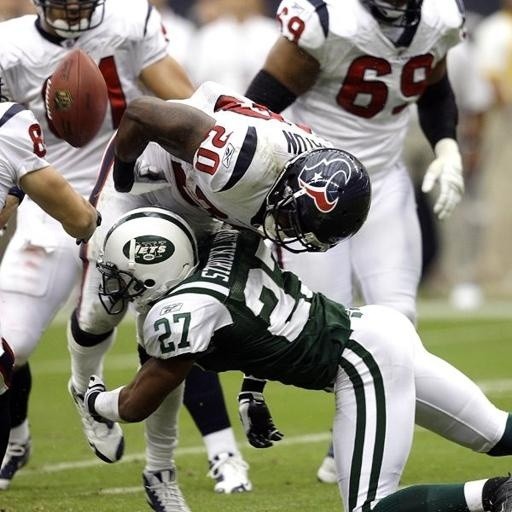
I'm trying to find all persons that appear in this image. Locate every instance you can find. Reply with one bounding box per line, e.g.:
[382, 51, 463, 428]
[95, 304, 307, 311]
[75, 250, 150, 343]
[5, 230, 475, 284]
[0, 99, 103, 472]
[69, 79, 373, 465]
[0, 0, 512, 316]
[240, 0, 467, 487]
[0, 0, 199, 491]
[87, 205, 512, 512]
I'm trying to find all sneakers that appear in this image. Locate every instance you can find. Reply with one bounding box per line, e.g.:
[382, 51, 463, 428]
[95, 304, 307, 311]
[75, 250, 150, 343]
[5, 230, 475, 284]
[315, 457, 341, 487]
[205, 448, 254, 496]
[488, 473, 511, 511]
[140, 462, 192, 512]
[65, 371, 127, 465]
[0, 430, 34, 492]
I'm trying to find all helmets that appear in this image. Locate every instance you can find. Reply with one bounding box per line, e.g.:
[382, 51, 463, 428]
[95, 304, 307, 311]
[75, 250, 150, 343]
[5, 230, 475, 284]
[95, 205, 202, 313]
[262, 145, 375, 257]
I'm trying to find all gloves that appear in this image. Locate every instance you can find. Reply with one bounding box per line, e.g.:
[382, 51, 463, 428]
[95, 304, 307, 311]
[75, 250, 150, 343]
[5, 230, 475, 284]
[421, 137, 468, 223]
[236, 378, 285, 449]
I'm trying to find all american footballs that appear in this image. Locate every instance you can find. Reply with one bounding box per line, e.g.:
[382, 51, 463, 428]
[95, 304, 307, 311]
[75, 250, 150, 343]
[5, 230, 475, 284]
[46, 49, 105, 147]
